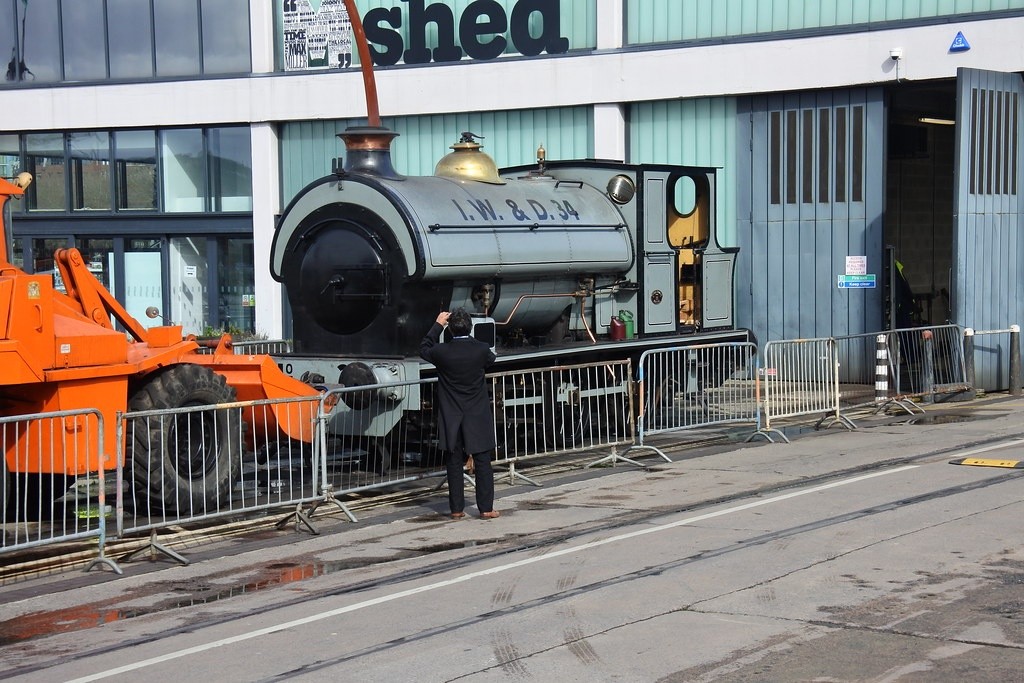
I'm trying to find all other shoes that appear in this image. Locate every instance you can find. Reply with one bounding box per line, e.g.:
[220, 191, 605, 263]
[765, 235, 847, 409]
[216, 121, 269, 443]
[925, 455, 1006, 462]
[480, 509, 499, 520]
[450, 512, 465, 519]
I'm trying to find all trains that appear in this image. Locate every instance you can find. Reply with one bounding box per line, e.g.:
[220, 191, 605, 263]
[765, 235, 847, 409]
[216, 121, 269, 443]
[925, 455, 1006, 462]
[260, 125, 757, 477]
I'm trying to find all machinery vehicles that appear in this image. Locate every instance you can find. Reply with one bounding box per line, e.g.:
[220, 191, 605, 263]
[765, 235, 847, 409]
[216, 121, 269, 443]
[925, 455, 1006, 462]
[0, 172, 346, 514]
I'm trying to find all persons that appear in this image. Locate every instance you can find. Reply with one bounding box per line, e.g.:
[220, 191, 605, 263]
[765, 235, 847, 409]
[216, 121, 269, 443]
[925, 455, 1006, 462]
[416, 305, 502, 523]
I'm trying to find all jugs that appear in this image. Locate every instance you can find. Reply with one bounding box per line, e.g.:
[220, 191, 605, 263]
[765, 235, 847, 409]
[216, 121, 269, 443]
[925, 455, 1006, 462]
[619, 310, 634, 339]
[611, 316, 626, 340]
